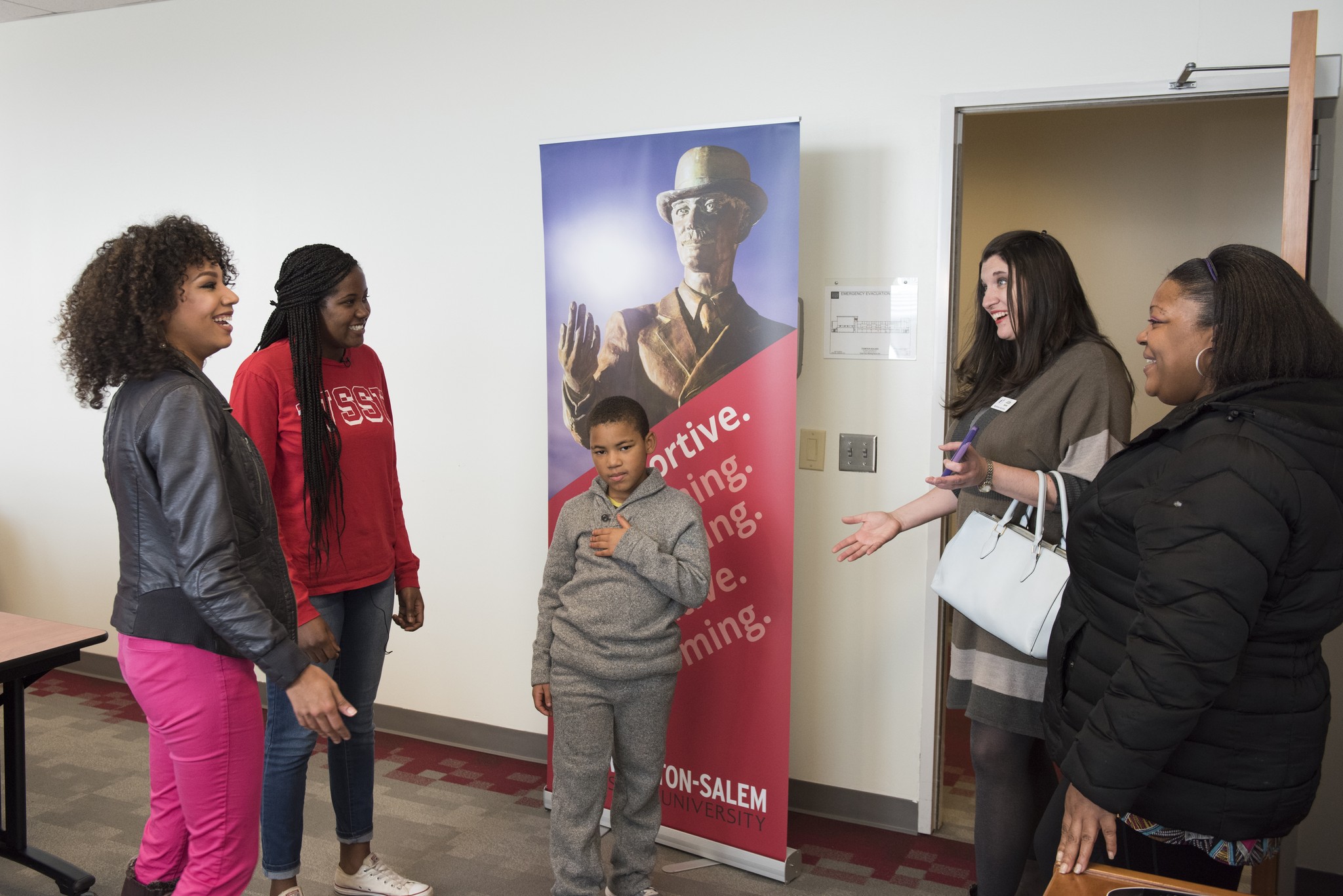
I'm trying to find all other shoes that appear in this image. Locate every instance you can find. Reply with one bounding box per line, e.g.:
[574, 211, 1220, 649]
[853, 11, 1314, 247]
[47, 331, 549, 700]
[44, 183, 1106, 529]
[605, 885, 660, 896]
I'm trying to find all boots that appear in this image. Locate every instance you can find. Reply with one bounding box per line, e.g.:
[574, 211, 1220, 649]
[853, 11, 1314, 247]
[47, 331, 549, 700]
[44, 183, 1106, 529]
[121, 856, 180, 896]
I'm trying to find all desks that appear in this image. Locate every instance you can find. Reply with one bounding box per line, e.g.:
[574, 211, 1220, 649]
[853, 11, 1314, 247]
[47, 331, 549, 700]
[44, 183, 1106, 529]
[0, 611, 109, 896]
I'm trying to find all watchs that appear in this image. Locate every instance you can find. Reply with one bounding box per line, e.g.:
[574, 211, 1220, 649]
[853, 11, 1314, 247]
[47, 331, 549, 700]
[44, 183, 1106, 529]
[976, 457, 994, 493]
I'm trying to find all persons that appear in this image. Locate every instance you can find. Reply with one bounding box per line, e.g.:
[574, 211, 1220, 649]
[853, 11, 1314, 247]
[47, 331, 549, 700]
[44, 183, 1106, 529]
[230, 245, 438, 896]
[531, 394, 710, 896]
[556, 147, 797, 451]
[832, 231, 1139, 896]
[1012, 243, 1343, 896]
[58, 215, 358, 896]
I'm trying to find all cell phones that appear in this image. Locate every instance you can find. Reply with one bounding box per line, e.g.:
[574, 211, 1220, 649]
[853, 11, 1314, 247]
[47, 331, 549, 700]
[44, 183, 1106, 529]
[941, 426, 979, 477]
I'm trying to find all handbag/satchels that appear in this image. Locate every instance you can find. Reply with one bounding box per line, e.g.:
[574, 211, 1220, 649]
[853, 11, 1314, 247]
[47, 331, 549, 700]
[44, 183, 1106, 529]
[931, 469, 1070, 660]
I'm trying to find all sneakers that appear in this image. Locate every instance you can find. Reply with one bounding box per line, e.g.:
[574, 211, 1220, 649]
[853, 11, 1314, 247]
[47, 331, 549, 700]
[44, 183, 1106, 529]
[334, 852, 434, 895]
[277, 886, 304, 896]
